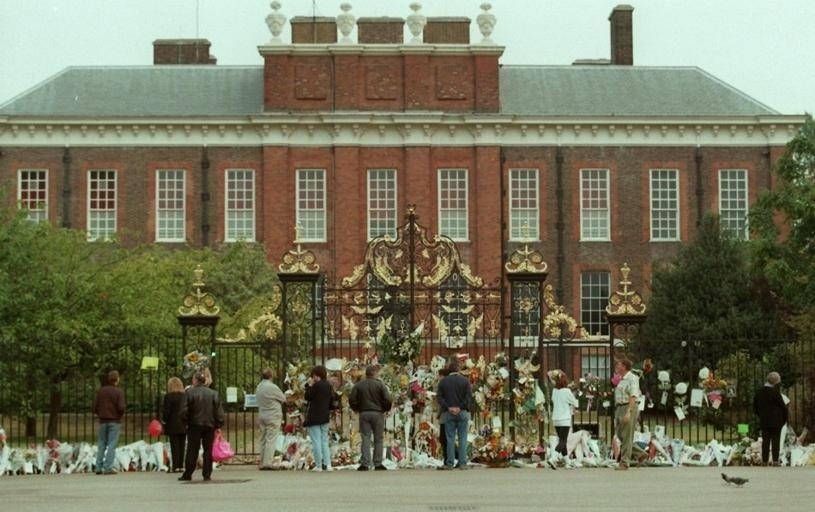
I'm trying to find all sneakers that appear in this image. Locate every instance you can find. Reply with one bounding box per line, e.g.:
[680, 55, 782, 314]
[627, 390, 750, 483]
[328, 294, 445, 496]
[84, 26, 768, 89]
[763, 462, 781, 466]
[311, 465, 331, 471]
[358, 465, 387, 470]
[259, 467, 275, 470]
[615, 453, 648, 470]
[96, 468, 118, 474]
[437, 464, 472, 469]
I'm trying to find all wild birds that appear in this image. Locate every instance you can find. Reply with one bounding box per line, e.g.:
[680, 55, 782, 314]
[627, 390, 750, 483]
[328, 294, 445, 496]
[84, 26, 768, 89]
[721, 473, 732, 485]
[729, 477, 749, 488]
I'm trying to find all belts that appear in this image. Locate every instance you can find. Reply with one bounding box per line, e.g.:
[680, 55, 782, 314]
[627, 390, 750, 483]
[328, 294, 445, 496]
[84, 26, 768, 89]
[617, 402, 630, 405]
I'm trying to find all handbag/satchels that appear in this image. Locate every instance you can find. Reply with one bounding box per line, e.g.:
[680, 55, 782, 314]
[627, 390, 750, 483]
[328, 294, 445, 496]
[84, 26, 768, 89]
[329, 391, 339, 409]
[212, 430, 234, 461]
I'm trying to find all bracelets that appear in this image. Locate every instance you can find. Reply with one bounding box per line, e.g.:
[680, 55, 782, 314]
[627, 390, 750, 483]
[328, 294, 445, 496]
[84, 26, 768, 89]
[627, 407, 632, 412]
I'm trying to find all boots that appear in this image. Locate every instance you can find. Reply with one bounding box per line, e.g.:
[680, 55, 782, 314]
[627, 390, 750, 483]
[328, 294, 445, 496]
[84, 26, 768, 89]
[548, 451, 574, 469]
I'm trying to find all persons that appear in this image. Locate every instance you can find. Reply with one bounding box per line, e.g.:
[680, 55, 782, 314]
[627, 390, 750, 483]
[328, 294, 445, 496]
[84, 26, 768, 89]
[174, 372, 224, 480]
[302, 366, 333, 470]
[159, 375, 186, 472]
[349, 365, 393, 471]
[433, 368, 462, 469]
[752, 371, 788, 466]
[609, 359, 643, 472]
[92, 369, 125, 475]
[254, 368, 286, 471]
[437, 362, 474, 470]
[547, 376, 580, 471]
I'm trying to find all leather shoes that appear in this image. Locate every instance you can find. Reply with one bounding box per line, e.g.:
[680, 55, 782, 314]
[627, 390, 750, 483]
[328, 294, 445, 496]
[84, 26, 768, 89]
[166, 467, 211, 481]
